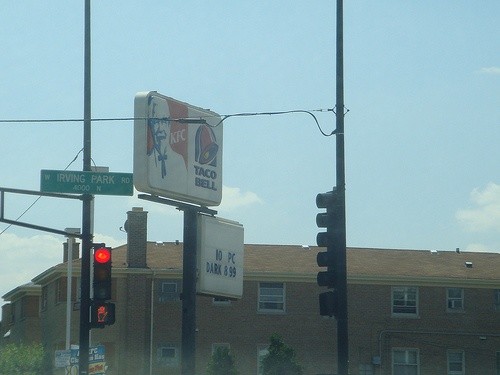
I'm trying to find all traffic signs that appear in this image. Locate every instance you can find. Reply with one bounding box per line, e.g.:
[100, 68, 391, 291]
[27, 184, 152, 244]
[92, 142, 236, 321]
[40, 169, 134, 196]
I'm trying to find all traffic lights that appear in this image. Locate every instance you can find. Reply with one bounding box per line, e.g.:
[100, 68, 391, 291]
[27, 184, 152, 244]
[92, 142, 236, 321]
[92, 245, 111, 300]
[316, 186, 349, 318]
[89, 303, 116, 326]
